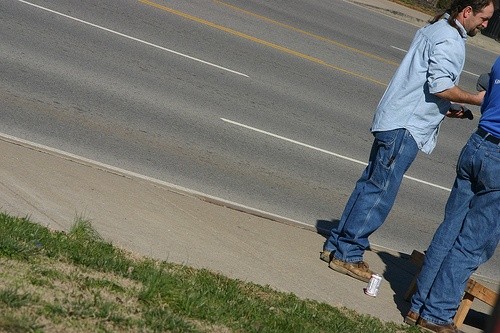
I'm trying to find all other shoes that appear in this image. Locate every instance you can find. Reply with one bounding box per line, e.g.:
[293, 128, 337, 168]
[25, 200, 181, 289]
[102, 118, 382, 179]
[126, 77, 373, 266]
[320, 248, 332, 263]
[329, 255, 374, 283]
[403, 309, 421, 327]
[416, 316, 464, 333]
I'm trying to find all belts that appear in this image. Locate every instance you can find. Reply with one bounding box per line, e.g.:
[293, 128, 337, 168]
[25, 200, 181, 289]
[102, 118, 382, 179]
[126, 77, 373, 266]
[476, 126, 500, 144]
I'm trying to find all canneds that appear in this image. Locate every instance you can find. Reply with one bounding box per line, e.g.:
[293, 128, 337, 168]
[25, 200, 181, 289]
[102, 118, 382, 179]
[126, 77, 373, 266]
[365, 273, 383, 297]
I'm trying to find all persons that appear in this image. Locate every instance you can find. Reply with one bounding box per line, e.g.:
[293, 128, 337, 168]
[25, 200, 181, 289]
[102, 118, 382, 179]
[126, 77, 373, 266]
[319, 0, 495, 284]
[404, 53, 500, 333]
